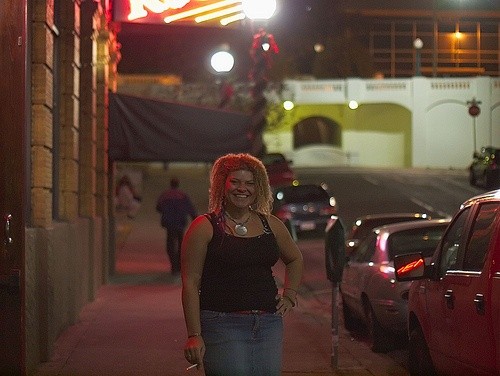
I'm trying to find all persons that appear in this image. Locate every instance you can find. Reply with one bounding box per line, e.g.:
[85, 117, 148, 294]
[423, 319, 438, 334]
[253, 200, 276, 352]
[181, 153, 304, 376]
[156, 176, 197, 274]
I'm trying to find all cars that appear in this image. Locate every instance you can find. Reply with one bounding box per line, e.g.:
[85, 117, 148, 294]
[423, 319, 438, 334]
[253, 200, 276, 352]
[394, 189, 500, 375]
[468, 146, 500, 188]
[260, 154, 339, 241]
[340, 214, 454, 353]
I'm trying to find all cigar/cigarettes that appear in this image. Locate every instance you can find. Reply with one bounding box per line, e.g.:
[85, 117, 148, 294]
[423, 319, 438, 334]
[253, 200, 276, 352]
[186, 364, 197, 371]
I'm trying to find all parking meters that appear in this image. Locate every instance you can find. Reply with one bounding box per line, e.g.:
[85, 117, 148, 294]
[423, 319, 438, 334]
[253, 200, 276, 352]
[324, 215, 346, 371]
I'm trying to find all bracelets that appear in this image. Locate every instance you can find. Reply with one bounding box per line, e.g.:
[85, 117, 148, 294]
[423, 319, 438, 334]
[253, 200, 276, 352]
[188, 333, 201, 338]
[284, 287, 298, 295]
[283, 296, 296, 308]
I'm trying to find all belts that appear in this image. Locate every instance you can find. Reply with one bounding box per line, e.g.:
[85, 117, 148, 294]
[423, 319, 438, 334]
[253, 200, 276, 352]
[235, 309, 270, 316]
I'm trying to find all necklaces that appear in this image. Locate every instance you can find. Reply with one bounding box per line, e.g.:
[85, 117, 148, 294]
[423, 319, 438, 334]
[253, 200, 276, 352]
[223, 209, 253, 236]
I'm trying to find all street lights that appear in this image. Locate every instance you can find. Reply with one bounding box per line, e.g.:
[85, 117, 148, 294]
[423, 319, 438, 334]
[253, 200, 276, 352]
[242, 0, 276, 156]
[211, 51, 234, 108]
